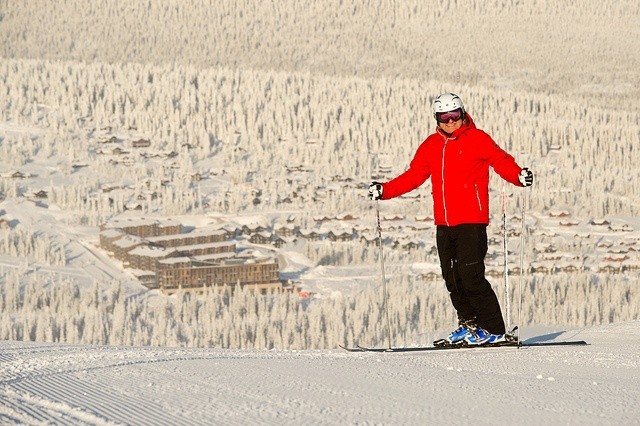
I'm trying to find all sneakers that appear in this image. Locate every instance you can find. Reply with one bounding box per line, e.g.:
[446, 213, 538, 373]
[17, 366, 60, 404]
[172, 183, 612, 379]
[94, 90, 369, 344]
[464, 329, 507, 346]
[446, 318, 481, 345]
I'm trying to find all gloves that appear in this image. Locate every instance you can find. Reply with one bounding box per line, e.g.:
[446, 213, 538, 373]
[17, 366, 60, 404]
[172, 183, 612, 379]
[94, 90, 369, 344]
[368, 183, 384, 200]
[518, 169, 533, 187]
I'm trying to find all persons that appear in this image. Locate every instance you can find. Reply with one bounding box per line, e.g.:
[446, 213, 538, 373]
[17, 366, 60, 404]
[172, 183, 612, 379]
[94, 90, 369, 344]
[367, 93, 532, 346]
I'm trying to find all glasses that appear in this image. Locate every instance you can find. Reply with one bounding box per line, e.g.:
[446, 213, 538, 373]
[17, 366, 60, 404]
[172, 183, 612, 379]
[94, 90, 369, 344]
[433, 106, 464, 123]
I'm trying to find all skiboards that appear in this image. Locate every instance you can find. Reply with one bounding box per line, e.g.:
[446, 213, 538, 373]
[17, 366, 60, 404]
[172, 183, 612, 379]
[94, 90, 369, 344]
[339, 341, 587, 352]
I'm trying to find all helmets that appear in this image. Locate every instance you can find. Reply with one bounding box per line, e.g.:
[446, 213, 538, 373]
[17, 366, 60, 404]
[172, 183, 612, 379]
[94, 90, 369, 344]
[433, 93, 465, 119]
